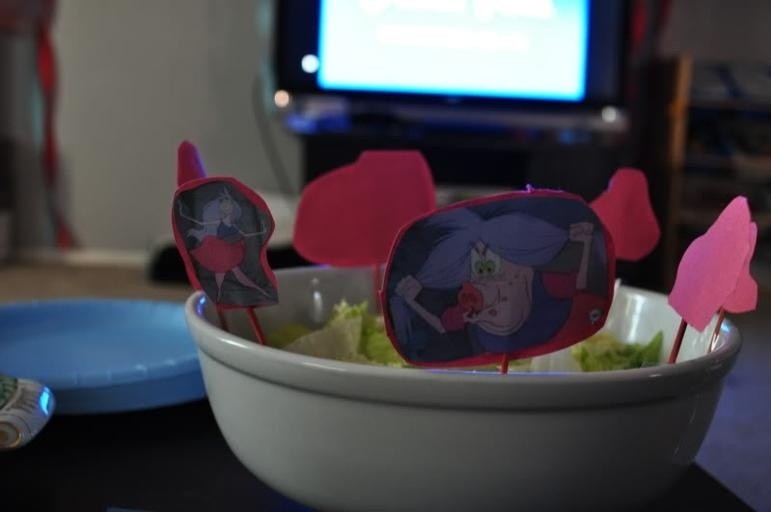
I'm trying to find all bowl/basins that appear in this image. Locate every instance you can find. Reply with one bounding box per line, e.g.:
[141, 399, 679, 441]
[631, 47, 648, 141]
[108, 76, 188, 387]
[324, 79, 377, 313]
[183, 263, 745, 512]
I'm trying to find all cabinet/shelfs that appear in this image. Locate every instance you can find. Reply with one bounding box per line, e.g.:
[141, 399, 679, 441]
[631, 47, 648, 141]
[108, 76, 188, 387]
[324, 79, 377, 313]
[655, 51, 771, 294]
[285, 115, 638, 258]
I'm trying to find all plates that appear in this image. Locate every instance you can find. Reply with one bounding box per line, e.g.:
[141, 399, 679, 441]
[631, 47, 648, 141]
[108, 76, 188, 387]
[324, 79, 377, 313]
[1, 297, 210, 412]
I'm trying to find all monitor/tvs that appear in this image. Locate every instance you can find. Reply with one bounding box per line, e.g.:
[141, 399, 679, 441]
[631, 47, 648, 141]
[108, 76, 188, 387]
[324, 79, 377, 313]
[274, 0, 623, 110]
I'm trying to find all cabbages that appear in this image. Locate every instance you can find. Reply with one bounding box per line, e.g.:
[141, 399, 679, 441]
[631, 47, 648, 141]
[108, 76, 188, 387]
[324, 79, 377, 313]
[271, 299, 664, 375]
